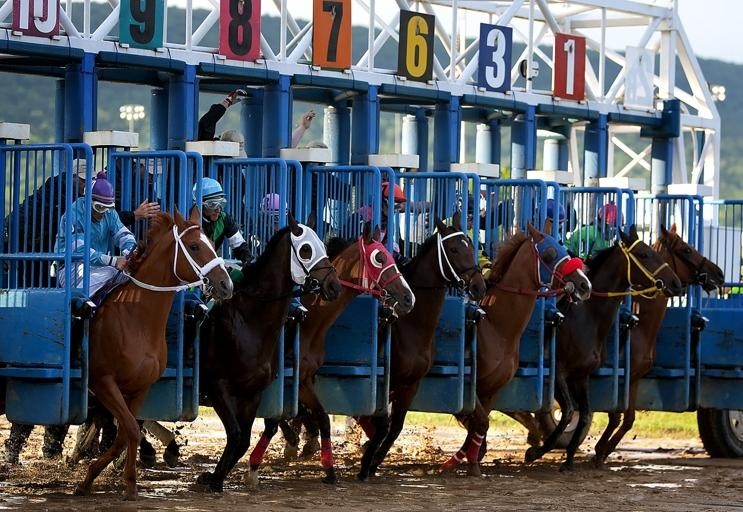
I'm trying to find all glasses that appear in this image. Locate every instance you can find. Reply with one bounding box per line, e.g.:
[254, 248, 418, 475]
[203, 197, 228, 211]
[92, 200, 116, 214]
[385, 198, 402, 213]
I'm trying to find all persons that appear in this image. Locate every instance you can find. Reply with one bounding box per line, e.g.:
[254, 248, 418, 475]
[0, 147, 167, 318]
[188, 179, 626, 326]
[199, 90, 355, 258]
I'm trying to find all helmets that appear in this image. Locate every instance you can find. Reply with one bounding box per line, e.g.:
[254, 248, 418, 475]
[382, 182, 407, 203]
[545, 198, 566, 223]
[191, 177, 227, 206]
[83, 171, 116, 203]
[598, 204, 625, 227]
[260, 193, 288, 216]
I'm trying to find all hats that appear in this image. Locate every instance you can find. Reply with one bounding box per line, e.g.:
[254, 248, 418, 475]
[72, 158, 97, 181]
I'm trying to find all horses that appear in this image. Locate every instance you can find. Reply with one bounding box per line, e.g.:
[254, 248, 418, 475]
[0, 204, 724, 502]
